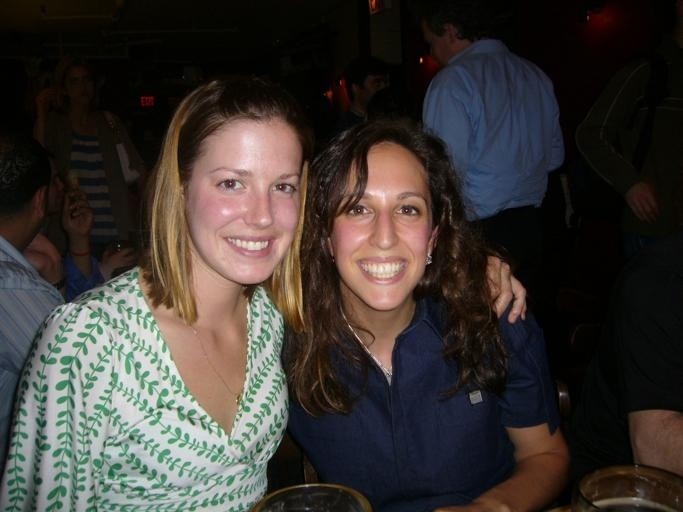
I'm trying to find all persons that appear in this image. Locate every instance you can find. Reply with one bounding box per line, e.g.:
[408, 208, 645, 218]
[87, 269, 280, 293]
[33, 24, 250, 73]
[0, 0, 682, 511]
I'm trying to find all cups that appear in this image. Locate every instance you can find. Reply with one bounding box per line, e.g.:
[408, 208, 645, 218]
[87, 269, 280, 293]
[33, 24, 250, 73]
[571, 464, 683, 512]
